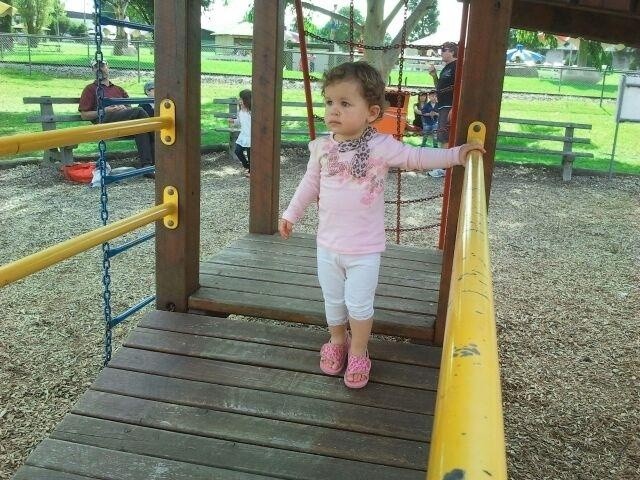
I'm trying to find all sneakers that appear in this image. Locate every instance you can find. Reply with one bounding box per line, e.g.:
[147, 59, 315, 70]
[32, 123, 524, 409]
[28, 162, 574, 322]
[244, 169, 250, 177]
[142, 164, 156, 179]
[427, 168, 446, 178]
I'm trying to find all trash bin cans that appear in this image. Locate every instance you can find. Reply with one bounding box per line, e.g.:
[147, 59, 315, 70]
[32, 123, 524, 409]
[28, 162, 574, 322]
[372, 92, 409, 141]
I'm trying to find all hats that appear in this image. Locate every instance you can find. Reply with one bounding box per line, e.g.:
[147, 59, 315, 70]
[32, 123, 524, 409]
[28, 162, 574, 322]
[144, 81, 156, 91]
[90, 56, 107, 66]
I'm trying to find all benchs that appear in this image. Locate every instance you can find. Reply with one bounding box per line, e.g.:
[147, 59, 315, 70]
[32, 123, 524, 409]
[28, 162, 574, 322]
[495, 116, 593, 181]
[23, 96, 154, 169]
[212, 98, 333, 160]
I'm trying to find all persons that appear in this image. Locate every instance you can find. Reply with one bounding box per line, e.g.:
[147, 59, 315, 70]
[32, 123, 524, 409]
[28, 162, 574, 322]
[137, 81, 156, 117]
[76, 56, 155, 179]
[277, 61, 488, 390]
[226, 89, 253, 177]
[422, 41, 459, 179]
[412, 91, 439, 130]
[420, 90, 440, 149]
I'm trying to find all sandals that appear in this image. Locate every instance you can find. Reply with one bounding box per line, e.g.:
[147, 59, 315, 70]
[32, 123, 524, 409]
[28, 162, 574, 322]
[320, 329, 350, 373]
[343, 350, 371, 390]
